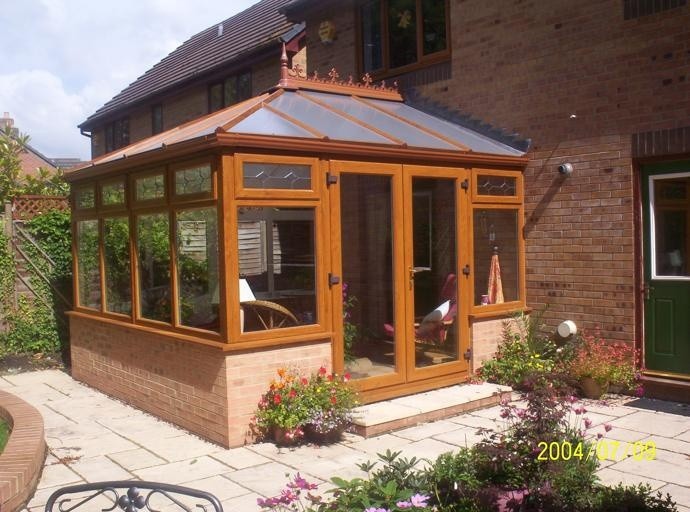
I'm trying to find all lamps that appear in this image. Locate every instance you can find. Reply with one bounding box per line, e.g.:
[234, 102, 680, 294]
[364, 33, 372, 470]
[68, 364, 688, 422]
[210, 278, 256, 333]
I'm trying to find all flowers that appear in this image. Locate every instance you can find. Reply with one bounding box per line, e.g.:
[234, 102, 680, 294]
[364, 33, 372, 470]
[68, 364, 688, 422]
[466, 304, 648, 402]
[248, 361, 362, 443]
[339, 280, 362, 367]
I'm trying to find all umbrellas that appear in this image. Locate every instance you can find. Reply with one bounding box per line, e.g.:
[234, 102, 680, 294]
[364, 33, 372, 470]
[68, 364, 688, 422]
[486, 245, 505, 305]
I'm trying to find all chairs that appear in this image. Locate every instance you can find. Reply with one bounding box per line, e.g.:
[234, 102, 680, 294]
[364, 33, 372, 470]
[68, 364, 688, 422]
[381, 272, 457, 363]
